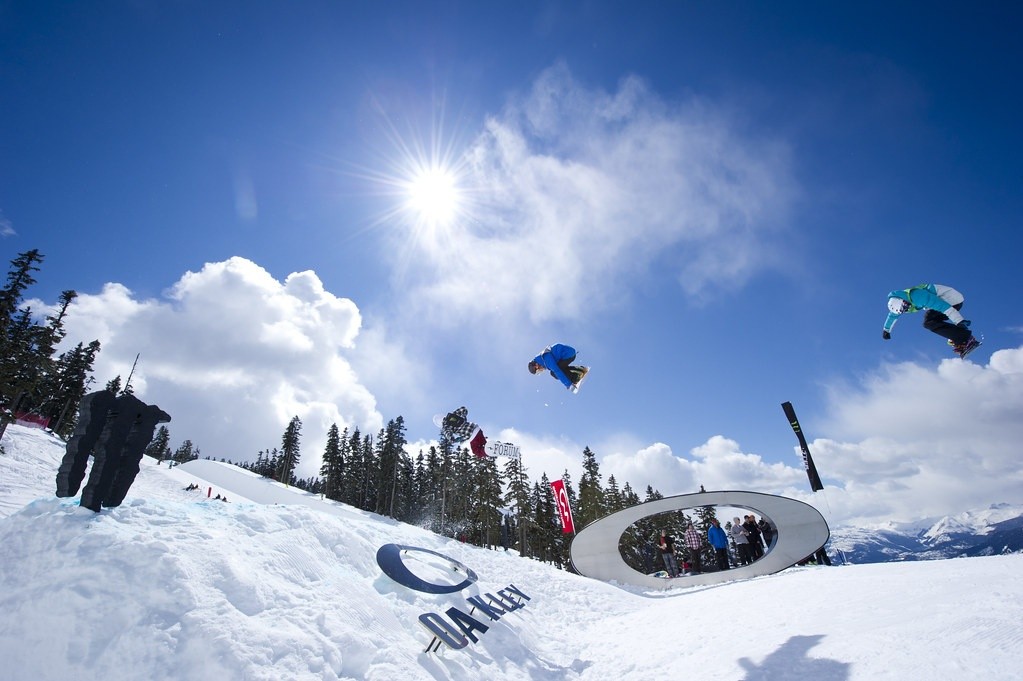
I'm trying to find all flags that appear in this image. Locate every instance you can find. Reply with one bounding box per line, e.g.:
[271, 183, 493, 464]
[551, 480, 573, 533]
[782, 401, 823, 492]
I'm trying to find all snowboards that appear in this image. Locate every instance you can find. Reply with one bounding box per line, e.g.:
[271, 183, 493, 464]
[946, 335, 983, 360]
[566, 366, 590, 390]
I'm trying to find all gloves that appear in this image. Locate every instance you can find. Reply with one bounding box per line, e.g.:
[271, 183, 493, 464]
[882, 331, 891, 340]
[570, 385, 578, 394]
[958, 320, 971, 329]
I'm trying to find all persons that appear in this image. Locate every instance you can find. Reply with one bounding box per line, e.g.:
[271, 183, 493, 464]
[882, 283, 974, 353]
[686, 523, 702, 575]
[797, 547, 831, 566]
[708, 518, 730, 571]
[658, 528, 679, 578]
[529, 343, 587, 392]
[731, 514, 773, 565]
[433, 406, 502, 460]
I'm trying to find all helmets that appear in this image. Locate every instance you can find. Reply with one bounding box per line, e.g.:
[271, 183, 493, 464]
[888, 297, 908, 315]
[528, 361, 537, 374]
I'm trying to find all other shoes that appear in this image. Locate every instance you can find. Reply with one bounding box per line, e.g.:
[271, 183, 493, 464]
[952, 337, 975, 354]
[572, 372, 582, 383]
[577, 366, 587, 372]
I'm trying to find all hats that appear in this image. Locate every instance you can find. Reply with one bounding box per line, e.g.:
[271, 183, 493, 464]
[744, 515, 750, 519]
